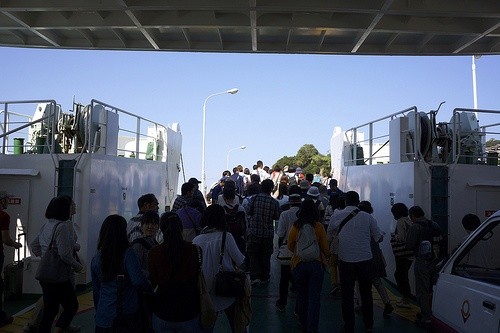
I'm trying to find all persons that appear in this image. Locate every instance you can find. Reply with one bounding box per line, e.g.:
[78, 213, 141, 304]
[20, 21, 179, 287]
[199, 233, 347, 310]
[172, 183, 194, 212]
[354, 201, 394, 316]
[207, 161, 347, 295]
[327, 191, 386, 333]
[247, 178, 282, 289]
[275, 194, 303, 320]
[189, 178, 207, 208]
[127, 193, 159, 247]
[0, 189, 23, 328]
[192, 204, 248, 333]
[137, 211, 203, 333]
[405, 206, 442, 319]
[288, 199, 329, 333]
[176, 200, 204, 243]
[24, 194, 84, 333]
[131, 211, 159, 258]
[90, 215, 142, 333]
[307, 186, 326, 224]
[390, 203, 413, 308]
[327, 179, 344, 200]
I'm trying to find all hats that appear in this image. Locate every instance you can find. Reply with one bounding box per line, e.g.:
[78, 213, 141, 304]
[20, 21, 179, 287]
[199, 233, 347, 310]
[188, 178, 201, 185]
[0, 191, 14, 199]
[285, 194, 303, 205]
[306, 186, 320, 196]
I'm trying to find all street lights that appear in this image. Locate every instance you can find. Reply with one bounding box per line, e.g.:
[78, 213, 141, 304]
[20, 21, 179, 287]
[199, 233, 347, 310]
[201, 88, 240, 199]
[226, 145, 247, 171]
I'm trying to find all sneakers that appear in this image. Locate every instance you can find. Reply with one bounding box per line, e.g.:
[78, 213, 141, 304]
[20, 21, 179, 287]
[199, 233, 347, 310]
[276, 299, 286, 311]
[54, 322, 80, 333]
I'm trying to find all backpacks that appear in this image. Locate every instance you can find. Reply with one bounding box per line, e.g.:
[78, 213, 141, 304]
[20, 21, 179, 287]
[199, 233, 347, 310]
[224, 205, 246, 237]
[294, 224, 319, 258]
[414, 220, 448, 268]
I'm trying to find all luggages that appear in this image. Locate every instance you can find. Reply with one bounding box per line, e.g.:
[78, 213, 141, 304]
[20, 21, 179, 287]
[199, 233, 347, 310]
[5, 233, 26, 304]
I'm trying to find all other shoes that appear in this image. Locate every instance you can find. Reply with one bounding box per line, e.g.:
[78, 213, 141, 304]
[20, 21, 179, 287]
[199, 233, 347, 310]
[419, 314, 431, 324]
[366, 325, 372, 332]
[354, 305, 362, 312]
[396, 301, 411, 308]
[383, 303, 394, 314]
[309, 329, 320, 333]
[251, 279, 262, 287]
[292, 311, 300, 319]
[341, 328, 348, 333]
[262, 280, 269, 285]
[0, 316, 14, 328]
[297, 321, 305, 329]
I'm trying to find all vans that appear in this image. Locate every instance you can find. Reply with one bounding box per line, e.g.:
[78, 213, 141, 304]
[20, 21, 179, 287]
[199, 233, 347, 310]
[430, 209, 500, 333]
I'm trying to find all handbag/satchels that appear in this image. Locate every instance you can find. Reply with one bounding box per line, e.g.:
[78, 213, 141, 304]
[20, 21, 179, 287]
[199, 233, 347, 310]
[200, 294, 218, 330]
[112, 306, 155, 333]
[390, 221, 408, 256]
[214, 270, 252, 333]
[276, 241, 292, 265]
[35, 222, 70, 282]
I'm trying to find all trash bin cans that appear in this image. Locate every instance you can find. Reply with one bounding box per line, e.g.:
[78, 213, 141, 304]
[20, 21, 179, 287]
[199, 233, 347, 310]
[146, 139, 163, 160]
[13, 138, 25, 154]
[37, 137, 48, 152]
